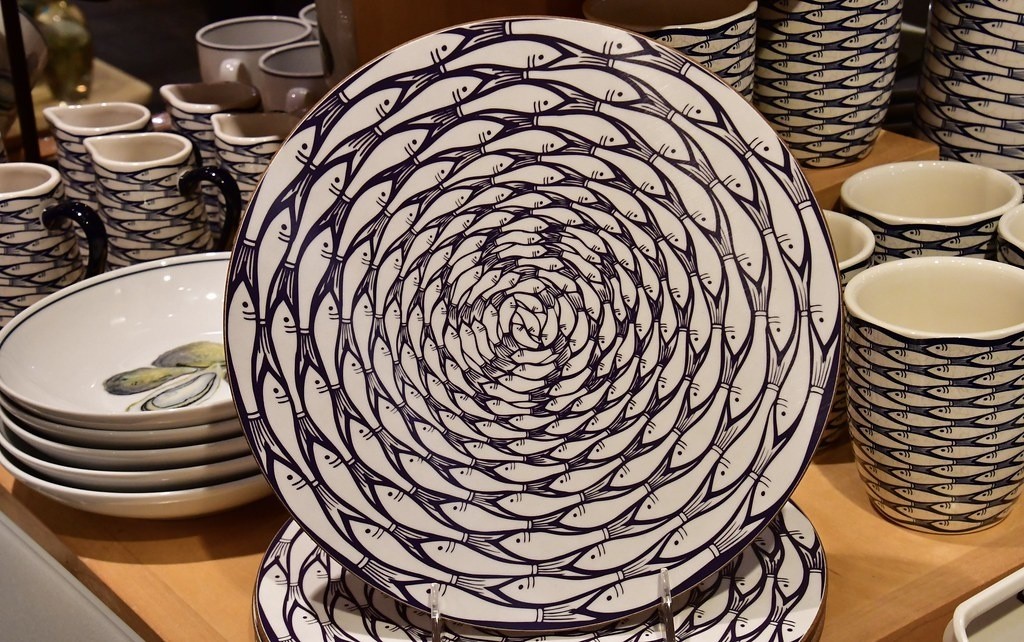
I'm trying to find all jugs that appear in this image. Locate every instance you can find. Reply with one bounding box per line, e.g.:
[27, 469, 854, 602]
[0, 81, 307, 320]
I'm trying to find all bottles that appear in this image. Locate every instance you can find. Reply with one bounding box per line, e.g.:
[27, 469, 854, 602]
[32, 0, 97, 103]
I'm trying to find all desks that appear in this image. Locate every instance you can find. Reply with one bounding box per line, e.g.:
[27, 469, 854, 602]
[798, 127, 941, 212]
[0, 428, 1024, 642]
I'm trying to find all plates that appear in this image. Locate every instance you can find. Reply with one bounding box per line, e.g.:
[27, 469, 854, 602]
[0, 248, 274, 521]
[247, 498, 830, 642]
[218, 14, 841, 633]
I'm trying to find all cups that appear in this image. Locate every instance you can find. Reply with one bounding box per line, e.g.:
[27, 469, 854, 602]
[844, 255, 1024, 535]
[755, 0, 906, 170]
[817, 206, 877, 451]
[838, 158, 1021, 264]
[997, 199, 1024, 268]
[195, 1, 325, 116]
[916, 0, 1023, 190]
[580, 0, 756, 104]
